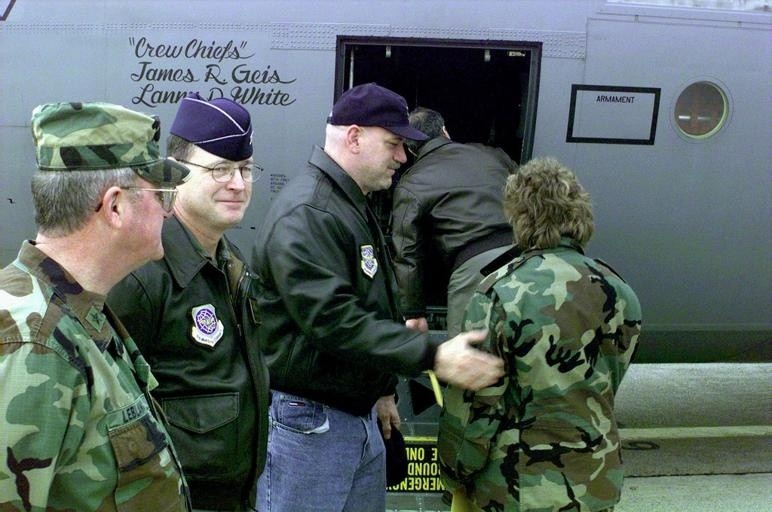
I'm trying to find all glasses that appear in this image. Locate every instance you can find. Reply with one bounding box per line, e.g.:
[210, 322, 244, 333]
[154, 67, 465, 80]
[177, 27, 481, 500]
[94, 186, 180, 213]
[177, 158, 265, 184]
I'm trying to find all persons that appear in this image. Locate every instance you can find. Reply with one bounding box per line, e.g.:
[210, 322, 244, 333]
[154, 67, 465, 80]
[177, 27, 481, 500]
[435, 154, 644, 511]
[386, 106, 524, 511]
[104, 91, 271, 511]
[248, 80, 508, 512]
[0, 100, 196, 512]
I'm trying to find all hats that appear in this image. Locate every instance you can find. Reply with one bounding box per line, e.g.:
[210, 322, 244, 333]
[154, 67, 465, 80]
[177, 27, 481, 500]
[326, 83, 430, 141]
[170, 92, 254, 162]
[30, 101, 192, 187]
